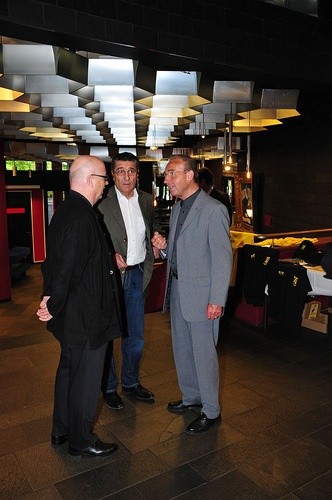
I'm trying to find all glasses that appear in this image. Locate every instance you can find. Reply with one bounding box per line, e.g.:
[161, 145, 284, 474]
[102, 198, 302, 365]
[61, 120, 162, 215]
[91, 174, 109, 180]
[163, 171, 176, 177]
[114, 170, 136, 176]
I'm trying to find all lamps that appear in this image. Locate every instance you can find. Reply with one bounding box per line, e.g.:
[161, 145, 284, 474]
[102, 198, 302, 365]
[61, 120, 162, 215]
[0, 41, 304, 161]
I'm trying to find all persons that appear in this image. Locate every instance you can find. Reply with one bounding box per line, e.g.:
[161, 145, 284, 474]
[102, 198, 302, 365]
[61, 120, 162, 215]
[97, 152, 159, 410]
[151, 154, 233, 434]
[37, 156, 128, 458]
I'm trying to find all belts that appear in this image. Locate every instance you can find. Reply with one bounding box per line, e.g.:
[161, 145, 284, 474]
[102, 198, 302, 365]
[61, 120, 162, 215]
[170, 272, 179, 280]
[125, 262, 144, 270]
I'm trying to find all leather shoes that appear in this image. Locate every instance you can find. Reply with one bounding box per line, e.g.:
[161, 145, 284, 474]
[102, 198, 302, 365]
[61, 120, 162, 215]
[68, 439, 120, 457]
[186, 412, 222, 436]
[167, 400, 203, 414]
[121, 383, 155, 401]
[50, 432, 66, 445]
[103, 392, 126, 410]
